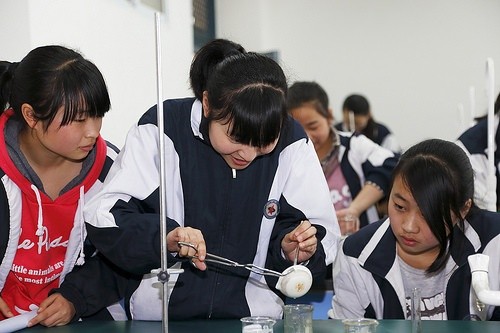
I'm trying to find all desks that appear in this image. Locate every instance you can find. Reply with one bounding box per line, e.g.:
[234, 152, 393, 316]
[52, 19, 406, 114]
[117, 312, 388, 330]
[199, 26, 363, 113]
[11, 319, 500, 333]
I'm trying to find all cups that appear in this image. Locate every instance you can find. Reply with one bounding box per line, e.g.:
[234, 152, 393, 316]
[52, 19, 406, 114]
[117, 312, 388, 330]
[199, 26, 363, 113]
[340, 216, 358, 236]
[240, 315, 276, 333]
[282, 304, 314, 333]
[342, 318, 379, 333]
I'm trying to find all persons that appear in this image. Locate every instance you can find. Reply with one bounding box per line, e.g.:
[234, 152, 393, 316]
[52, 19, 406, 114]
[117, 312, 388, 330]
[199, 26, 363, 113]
[0, 46, 145, 333]
[326, 138, 500, 322]
[329, 93, 392, 152]
[276, 75, 398, 246]
[452, 92, 500, 220]
[82, 40, 346, 328]
